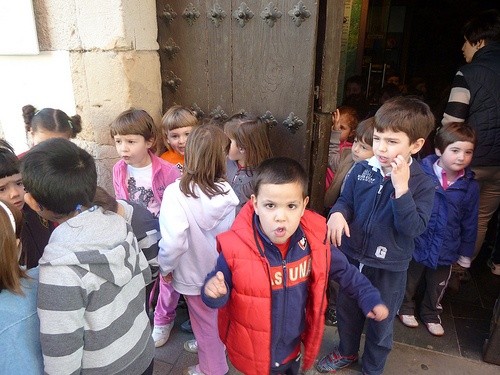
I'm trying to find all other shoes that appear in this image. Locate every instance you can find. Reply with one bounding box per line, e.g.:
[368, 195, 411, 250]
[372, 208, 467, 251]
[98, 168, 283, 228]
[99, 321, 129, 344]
[445, 272, 464, 299]
[326, 308, 338, 327]
[486, 259, 500, 276]
[181, 321, 192, 332]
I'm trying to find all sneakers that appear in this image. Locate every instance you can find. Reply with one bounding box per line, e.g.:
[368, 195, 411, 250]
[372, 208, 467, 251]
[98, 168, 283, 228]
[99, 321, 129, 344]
[396, 309, 418, 327]
[425, 323, 444, 335]
[151, 321, 174, 347]
[316, 349, 359, 373]
[184, 340, 198, 353]
[182, 366, 199, 375]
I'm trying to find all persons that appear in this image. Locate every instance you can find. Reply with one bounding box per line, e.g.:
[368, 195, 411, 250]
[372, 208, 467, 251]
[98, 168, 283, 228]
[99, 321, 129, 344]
[109, 108, 183, 347]
[321, 108, 375, 323]
[216, 113, 262, 212]
[1, 138, 46, 270]
[15, 104, 82, 159]
[151, 105, 199, 173]
[0, 199, 47, 375]
[202, 157, 390, 375]
[440, 23, 499, 274]
[91, 184, 161, 320]
[162, 124, 239, 374]
[19, 136, 155, 375]
[398, 120, 480, 336]
[316, 99, 434, 375]
[325, 111, 357, 189]
